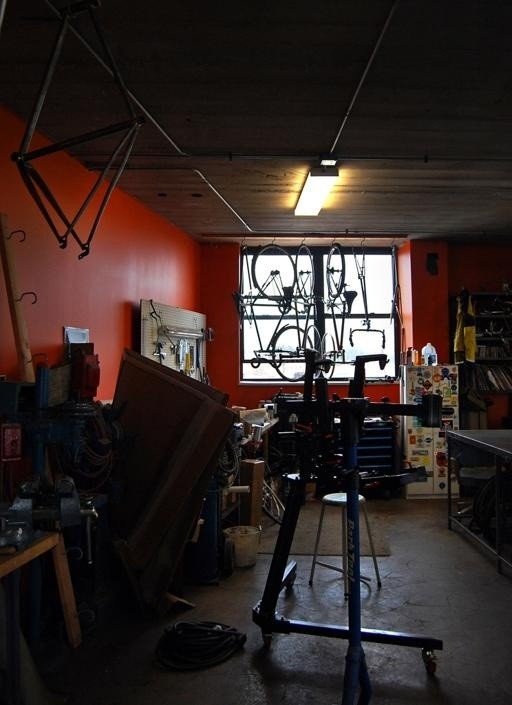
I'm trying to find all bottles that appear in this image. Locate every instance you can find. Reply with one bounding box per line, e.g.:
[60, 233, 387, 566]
[412, 347, 418, 364]
[428, 357, 433, 365]
[406, 347, 412, 366]
[420, 354, 425, 366]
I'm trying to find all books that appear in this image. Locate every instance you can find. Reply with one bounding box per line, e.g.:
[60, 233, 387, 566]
[456, 344, 512, 392]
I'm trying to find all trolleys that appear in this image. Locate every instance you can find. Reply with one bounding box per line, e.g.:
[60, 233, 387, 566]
[246, 343, 469, 704]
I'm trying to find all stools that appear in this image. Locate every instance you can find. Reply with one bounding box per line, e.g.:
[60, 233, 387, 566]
[306, 493, 386, 598]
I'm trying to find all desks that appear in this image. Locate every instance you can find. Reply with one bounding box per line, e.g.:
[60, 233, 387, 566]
[240, 416, 280, 474]
[446, 427, 512, 576]
[0, 529, 81, 647]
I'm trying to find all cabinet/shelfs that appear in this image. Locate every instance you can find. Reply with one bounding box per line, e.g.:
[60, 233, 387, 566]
[335, 419, 396, 500]
[454, 291, 512, 394]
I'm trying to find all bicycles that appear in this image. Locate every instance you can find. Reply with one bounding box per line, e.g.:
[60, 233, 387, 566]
[229, 239, 358, 384]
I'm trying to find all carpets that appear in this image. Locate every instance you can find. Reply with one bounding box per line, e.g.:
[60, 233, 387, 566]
[253, 499, 393, 558]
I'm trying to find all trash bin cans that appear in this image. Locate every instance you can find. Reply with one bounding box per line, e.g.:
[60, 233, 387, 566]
[223, 526, 260, 569]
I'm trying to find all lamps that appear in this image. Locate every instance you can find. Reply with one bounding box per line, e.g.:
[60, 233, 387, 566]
[294, 158, 339, 220]
[157, 322, 203, 341]
